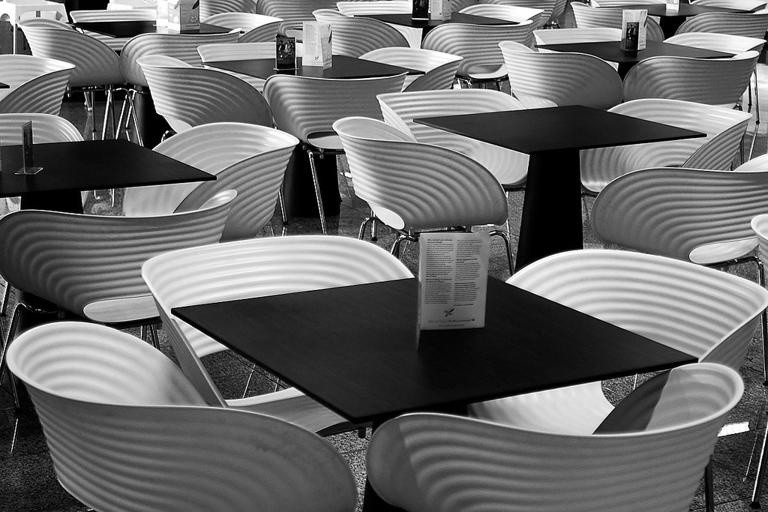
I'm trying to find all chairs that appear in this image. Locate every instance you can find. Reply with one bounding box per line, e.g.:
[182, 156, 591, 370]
[1, 188, 241, 459]
[0, 0, 768, 275]
[365, 362, 745, 512]
[503, 249, 768, 512]
[142, 234, 416, 433]
[7, 321, 362, 509]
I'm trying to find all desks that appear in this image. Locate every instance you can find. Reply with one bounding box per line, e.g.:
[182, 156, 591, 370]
[171, 274, 700, 424]
